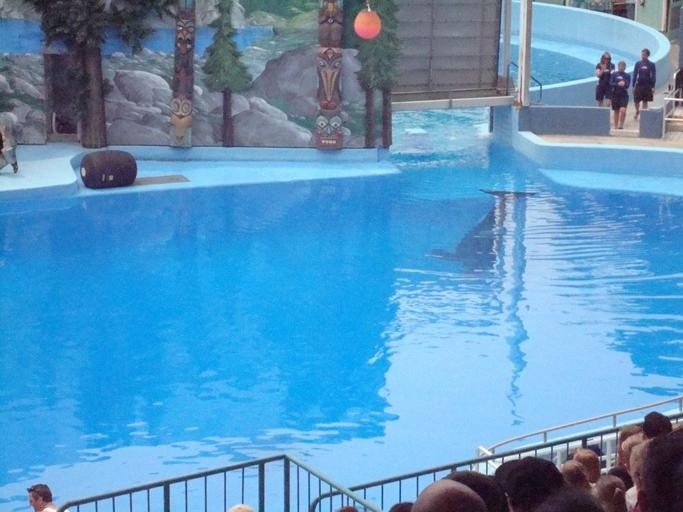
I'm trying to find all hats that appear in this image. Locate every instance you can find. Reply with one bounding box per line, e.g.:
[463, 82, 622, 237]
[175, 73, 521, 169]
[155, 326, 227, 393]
[643, 410, 672, 436]
[494, 456, 564, 505]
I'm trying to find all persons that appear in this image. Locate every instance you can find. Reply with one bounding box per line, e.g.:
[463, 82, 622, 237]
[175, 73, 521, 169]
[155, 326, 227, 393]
[609, 61, 630, 129]
[27, 484, 70, 512]
[337, 414, 681, 511]
[228, 504, 255, 512]
[595, 52, 615, 107]
[632, 49, 656, 118]
